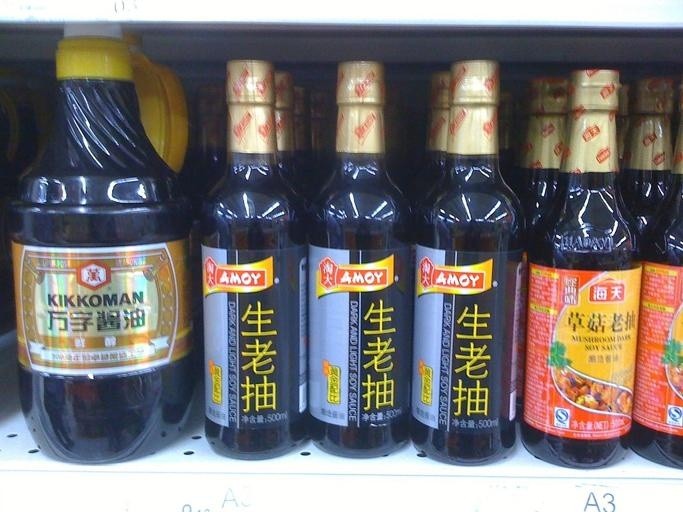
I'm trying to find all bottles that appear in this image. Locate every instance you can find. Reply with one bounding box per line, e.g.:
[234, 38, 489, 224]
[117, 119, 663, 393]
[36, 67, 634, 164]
[6, 20, 683, 469]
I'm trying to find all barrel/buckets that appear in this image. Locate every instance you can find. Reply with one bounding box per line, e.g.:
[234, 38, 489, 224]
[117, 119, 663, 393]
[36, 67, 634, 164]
[6, 21, 196, 465]
